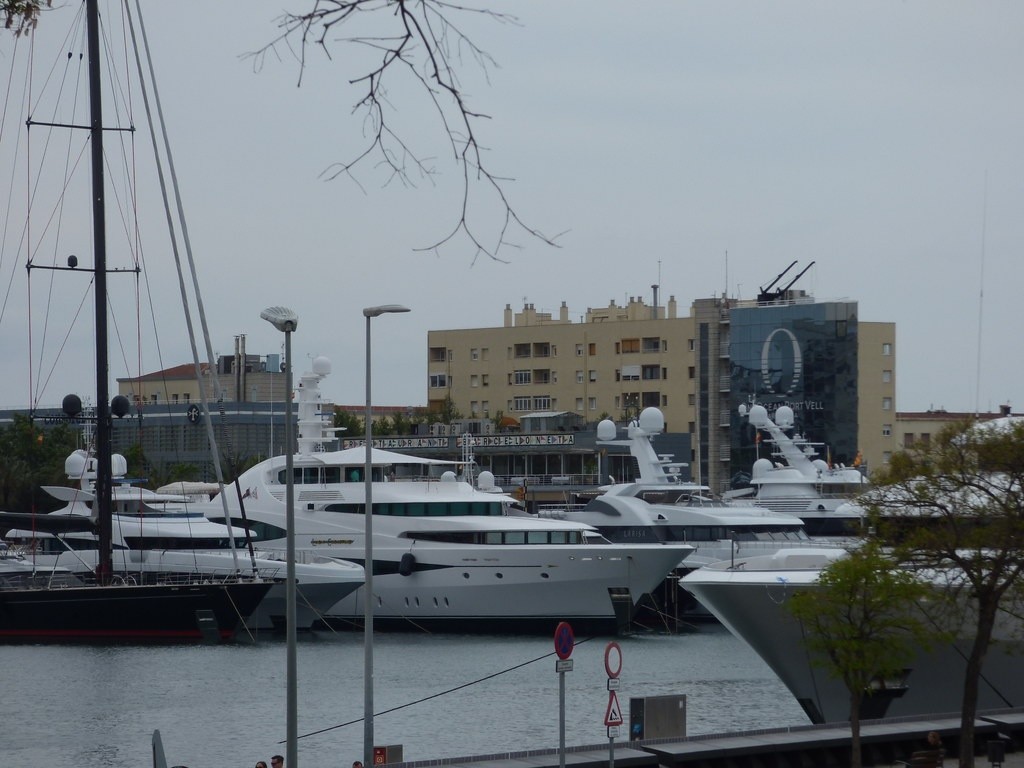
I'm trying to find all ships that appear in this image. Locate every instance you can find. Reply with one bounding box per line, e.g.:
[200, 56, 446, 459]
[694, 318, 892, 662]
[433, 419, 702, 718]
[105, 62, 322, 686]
[203, 445, 696, 624]
[566, 406, 1024, 725]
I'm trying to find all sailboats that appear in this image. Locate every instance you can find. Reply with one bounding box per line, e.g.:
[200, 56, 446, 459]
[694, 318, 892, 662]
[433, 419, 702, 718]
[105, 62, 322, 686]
[0, 0, 370, 638]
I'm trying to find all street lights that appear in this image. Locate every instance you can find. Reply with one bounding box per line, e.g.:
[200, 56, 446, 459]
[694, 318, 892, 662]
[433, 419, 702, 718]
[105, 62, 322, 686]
[364, 303, 412, 768]
[260, 305, 300, 767]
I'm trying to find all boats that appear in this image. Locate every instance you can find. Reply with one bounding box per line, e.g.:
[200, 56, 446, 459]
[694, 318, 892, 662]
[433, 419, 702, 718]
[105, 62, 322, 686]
[0, 449, 365, 636]
